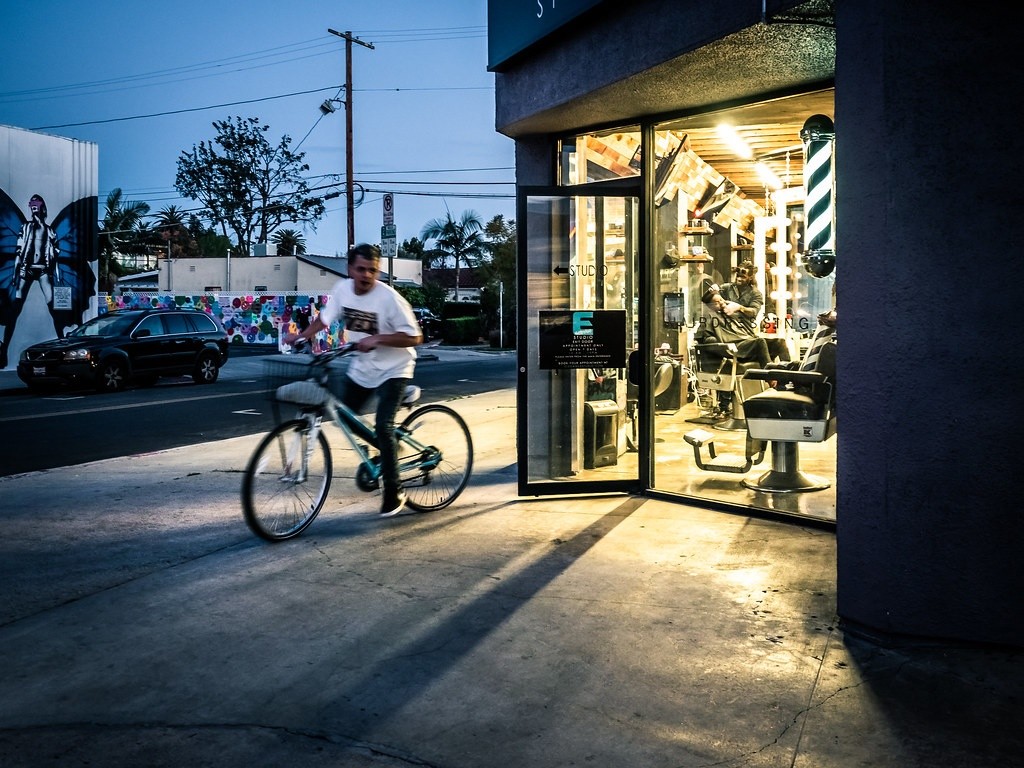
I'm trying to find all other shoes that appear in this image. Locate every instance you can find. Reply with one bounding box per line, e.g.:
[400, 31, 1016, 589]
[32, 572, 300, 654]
[712, 409, 727, 420]
[769, 380, 778, 388]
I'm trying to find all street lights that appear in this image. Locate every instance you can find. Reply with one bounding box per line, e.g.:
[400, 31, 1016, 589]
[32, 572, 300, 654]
[318, 99, 356, 276]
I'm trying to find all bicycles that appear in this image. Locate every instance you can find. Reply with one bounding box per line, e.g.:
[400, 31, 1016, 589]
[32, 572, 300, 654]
[239, 337, 472, 544]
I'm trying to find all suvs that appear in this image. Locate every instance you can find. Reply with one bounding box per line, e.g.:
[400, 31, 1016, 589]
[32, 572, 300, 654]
[15, 305, 231, 395]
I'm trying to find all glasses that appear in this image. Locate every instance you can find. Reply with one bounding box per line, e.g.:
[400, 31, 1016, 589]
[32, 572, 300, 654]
[736, 267, 747, 274]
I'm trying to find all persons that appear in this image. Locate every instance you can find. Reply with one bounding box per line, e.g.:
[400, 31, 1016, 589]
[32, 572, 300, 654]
[282, 242, 422, 518]
[711, 260, 764, 422]
[701, 290, 803, 389]
[816, 310, 837, 329]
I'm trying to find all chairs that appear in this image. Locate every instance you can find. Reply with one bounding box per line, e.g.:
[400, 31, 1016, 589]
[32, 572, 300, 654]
[683, 341, 837, 494]
[685, 333, 767, 432]
[626, 362, 674, 453]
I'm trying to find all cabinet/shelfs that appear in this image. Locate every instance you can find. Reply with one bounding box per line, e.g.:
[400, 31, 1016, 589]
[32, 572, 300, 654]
[731, 248, 754, 272]
[605, 232, 625, 264]
[678, 231, 713, 263]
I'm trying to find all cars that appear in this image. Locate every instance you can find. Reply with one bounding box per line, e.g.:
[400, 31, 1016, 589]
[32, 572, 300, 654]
[412, 307, 441, 344]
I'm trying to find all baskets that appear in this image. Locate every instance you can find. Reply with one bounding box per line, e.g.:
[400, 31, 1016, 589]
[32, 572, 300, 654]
[260, 359, 337, 414]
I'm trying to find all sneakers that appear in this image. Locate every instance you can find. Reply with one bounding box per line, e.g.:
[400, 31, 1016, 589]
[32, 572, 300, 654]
[378, 483, 410, 519]
